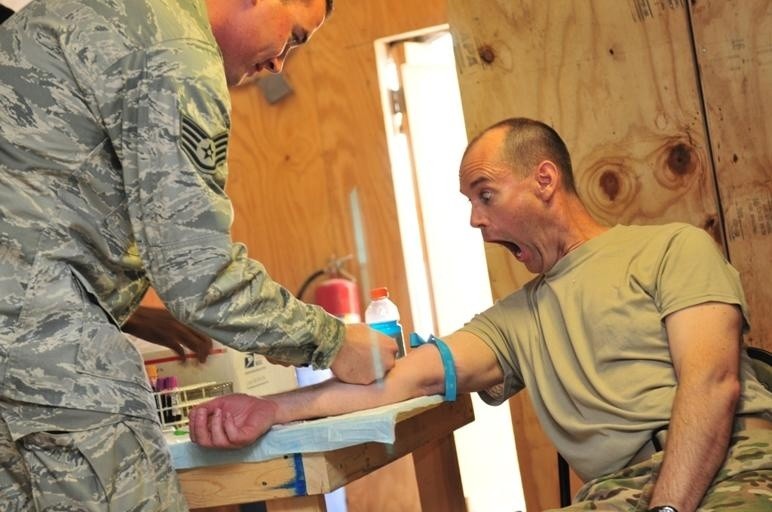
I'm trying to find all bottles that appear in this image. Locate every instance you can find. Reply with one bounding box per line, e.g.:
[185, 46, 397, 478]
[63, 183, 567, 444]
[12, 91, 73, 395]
[313, 277, 362, 327]
[364, 289, 405, 359]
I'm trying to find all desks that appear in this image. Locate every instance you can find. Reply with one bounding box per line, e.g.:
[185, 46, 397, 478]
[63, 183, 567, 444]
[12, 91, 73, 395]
[162, 393, 475, 511]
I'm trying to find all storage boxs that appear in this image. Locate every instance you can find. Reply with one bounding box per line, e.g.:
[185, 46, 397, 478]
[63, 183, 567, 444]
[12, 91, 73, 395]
[144, 341, 298, 396]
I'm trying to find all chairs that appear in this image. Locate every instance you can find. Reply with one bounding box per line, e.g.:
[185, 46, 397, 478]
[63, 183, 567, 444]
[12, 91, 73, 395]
[558, 348, 772, 507]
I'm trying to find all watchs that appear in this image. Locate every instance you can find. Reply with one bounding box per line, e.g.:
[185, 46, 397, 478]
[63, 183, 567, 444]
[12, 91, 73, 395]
[647, 505, 676, 512]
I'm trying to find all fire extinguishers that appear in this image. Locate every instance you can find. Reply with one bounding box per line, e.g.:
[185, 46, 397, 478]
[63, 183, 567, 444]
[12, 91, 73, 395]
[297, 249, 362, 325]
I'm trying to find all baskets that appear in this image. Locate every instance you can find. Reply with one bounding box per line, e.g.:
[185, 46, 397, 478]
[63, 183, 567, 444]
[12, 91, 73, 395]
[152, 382, 216, 433]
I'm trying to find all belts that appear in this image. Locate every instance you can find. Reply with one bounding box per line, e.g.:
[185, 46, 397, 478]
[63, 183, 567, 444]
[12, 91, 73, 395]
[625, 414, 771, 466]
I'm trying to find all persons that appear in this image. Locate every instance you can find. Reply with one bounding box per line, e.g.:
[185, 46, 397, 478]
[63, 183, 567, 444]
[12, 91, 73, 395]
[188, 117, 772, 512]
[0, 0, 400, 512]
